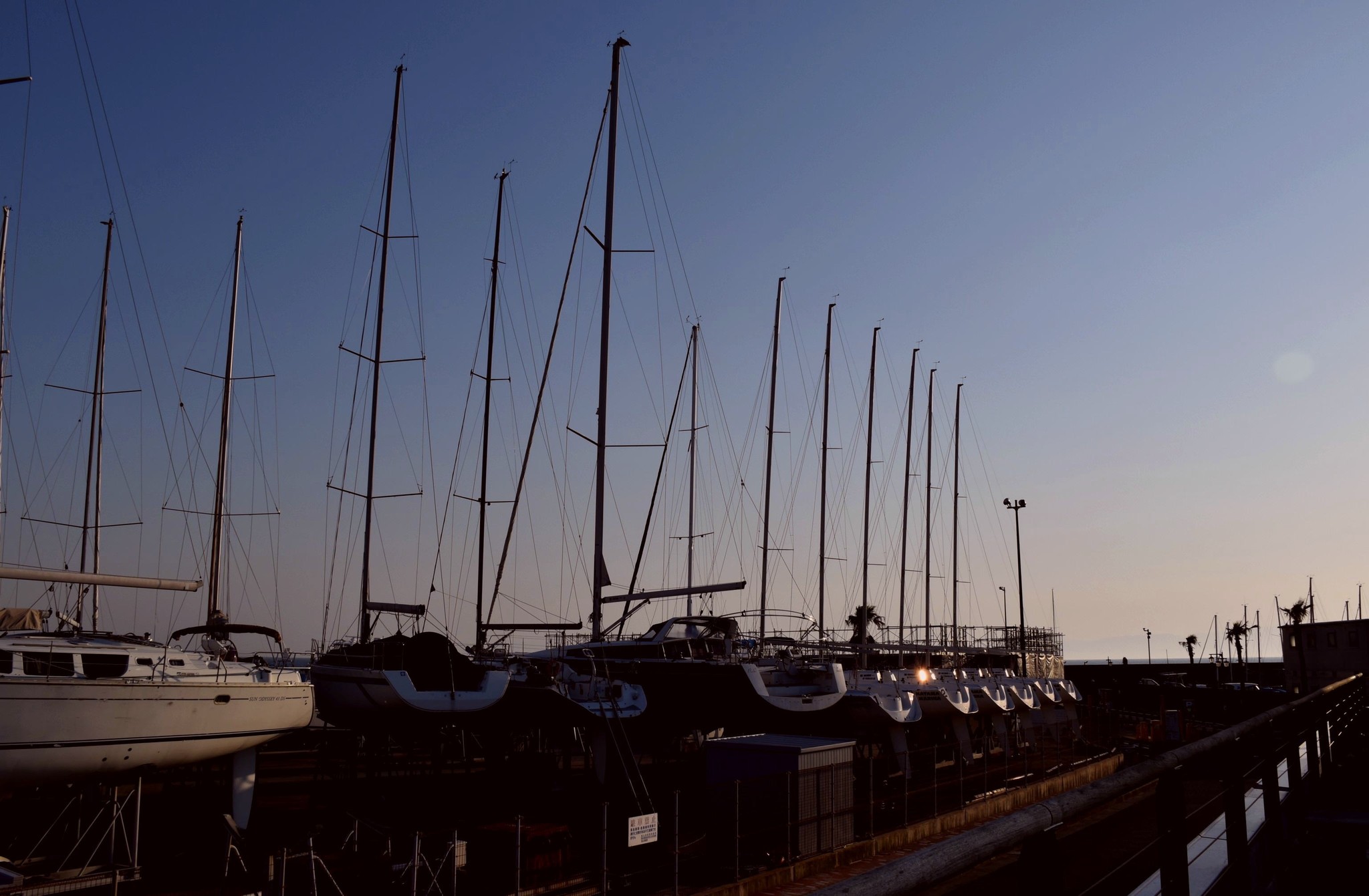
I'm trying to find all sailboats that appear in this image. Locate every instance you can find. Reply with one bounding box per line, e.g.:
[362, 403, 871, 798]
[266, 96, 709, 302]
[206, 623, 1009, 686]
[0, 30, 1081, 789]
[0, 202, 318, 779]
